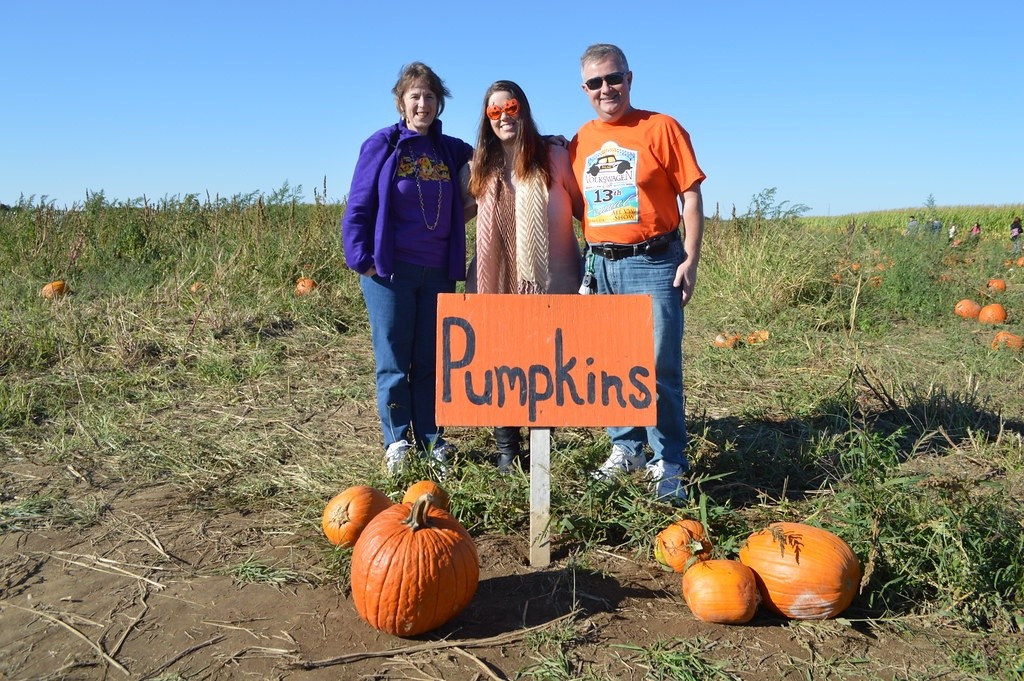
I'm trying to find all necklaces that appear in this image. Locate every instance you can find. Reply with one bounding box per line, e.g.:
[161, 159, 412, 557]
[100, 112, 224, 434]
[407, 140, 443, 229]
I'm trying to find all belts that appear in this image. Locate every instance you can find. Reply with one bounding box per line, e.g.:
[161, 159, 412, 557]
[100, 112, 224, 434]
[586, 229, 679, 261]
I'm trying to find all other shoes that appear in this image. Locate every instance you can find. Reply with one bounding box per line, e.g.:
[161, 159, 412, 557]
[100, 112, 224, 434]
[386, 440, 413, 475]
[416, 441, 458, 472]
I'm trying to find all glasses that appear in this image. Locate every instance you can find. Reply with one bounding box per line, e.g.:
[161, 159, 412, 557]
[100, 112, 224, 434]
[486, 98, 520, 120]
[585, 72, 628, 90]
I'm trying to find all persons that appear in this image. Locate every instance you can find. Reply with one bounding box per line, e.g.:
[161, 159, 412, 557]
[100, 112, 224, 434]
[342, 61, 571, 480]
[462, 79, 586, 472]
[1011, 217, 1023, 241]
[904, 216, 956, 244]
[567, 44, 707, 505]
[972, 222, 980, 236]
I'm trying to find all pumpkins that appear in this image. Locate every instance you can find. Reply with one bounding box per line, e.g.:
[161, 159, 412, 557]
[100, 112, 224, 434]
[294, 279, 315, 297]
[833, 240, 1024, 352]
[191, 282, 203, 292]
[322, 480, 479, 636]
[715, 329, 769, 347]
[41, 281, 67, 299]
[655, 520, 859, 624]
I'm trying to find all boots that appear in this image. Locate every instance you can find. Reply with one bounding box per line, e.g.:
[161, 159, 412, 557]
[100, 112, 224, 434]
[495, 426, 521, 471]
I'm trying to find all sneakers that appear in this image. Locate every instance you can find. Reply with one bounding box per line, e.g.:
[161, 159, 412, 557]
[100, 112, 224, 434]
[592, 444, 646, 480]
[643, 459, 687, 507]
